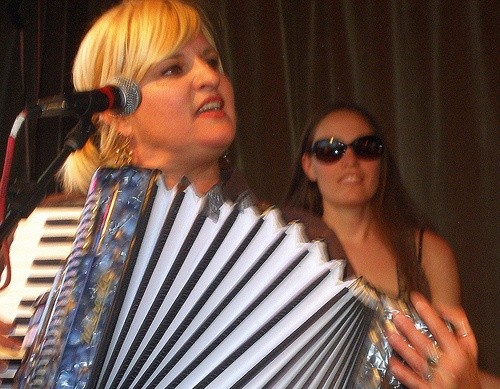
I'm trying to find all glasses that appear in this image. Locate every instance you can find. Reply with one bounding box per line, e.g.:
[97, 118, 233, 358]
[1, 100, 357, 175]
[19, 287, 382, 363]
[308, 136, 384, 164]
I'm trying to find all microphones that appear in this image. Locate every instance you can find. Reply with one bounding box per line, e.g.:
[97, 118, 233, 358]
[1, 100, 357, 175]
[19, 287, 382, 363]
[25, 77, 141, 121]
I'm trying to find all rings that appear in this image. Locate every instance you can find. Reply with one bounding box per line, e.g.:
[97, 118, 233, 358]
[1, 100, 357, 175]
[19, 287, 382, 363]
[424, 342, 440, 365]
[422, 365, 433, 381]
[451, 318, 470, 337]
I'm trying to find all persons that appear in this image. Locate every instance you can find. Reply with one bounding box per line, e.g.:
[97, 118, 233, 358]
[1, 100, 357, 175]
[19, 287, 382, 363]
[2, 0, 500, 389]
[277, 100, 459, 311]
